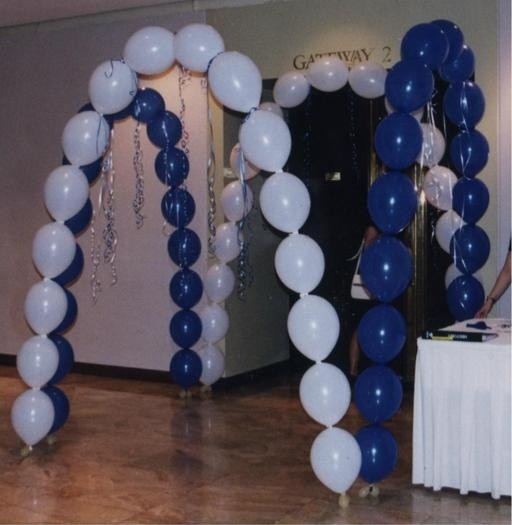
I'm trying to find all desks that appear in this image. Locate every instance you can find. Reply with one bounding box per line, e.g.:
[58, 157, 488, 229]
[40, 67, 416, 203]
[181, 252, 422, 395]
[410, 316, 512, 501]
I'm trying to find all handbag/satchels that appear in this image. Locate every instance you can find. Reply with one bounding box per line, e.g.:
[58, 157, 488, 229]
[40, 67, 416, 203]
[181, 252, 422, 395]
[346, 238, 373, 300]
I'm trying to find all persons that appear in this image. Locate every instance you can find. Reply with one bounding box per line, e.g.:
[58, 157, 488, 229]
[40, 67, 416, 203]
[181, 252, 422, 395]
[475, 236, 511, 326]
